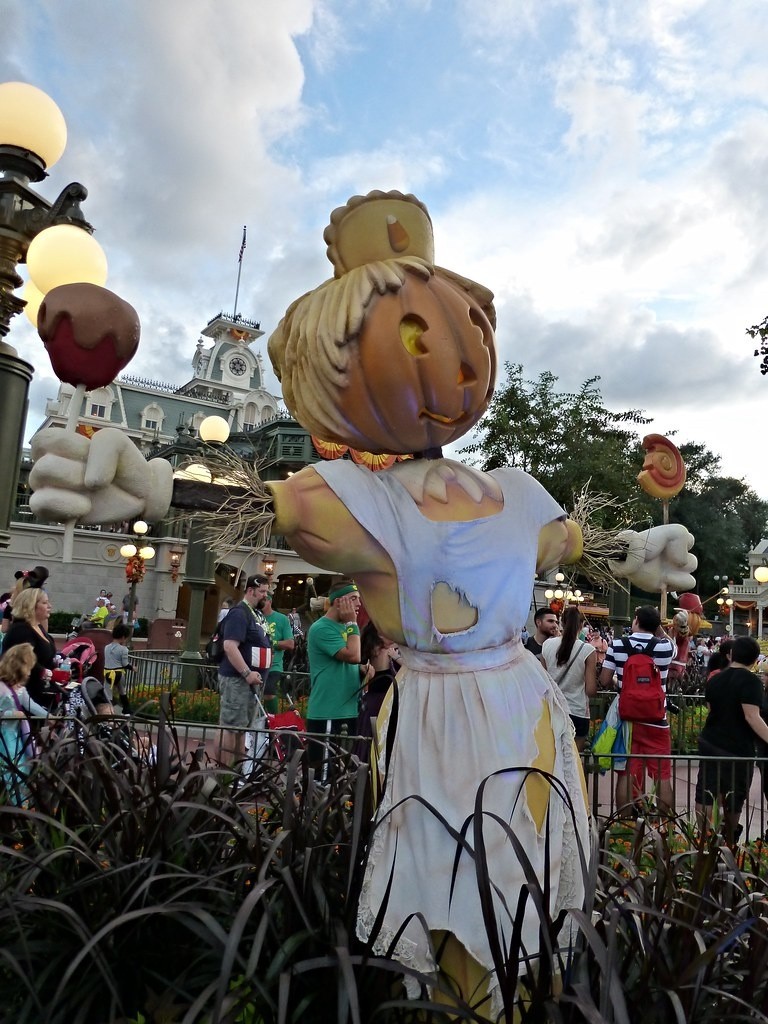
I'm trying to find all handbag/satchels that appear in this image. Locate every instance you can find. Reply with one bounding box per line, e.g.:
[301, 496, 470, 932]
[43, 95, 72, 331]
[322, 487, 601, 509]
[20, 714, 35, 753]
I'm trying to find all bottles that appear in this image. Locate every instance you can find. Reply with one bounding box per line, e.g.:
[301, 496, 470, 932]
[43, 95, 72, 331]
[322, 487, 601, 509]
[55, 656, 71, 686]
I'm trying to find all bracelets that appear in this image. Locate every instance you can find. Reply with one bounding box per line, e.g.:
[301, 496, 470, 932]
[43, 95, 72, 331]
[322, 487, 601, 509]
[242, 669, 251, 677]
[347, 625, 360, 636]
[395, 656, 401, 661]
[346, 621, 357, 627]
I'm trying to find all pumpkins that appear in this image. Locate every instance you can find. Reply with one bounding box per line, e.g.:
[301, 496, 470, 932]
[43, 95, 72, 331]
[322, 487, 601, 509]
[268, 264, 497, 447]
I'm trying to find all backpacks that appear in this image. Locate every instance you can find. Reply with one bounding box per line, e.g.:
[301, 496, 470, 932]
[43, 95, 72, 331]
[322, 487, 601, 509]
[618, 636, 666, 723]
[205, 606, 247, 664]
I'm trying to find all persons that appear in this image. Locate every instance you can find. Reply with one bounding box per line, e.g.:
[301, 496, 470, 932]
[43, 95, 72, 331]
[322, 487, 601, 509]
[211, 570, 405, 787]
[29, 193, 699, 1024]
[521, 605, 768, 841]
[0, 566, 139, 851]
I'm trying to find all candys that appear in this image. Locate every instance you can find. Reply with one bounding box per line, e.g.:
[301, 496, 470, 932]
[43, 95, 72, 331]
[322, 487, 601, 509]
[636, 433, 688, 501]
[37, 280, 141, 391]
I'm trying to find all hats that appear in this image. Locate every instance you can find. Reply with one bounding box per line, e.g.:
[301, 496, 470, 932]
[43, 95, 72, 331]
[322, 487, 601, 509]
[592, 628, 599, 632]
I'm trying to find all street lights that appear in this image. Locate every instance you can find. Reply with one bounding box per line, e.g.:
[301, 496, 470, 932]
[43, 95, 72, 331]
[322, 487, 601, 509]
[715, 575, 728, 596]
[118, 521, 156, 652]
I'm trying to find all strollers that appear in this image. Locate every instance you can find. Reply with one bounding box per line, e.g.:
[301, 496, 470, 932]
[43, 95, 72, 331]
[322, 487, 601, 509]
[57, 637, 99, 681]
[49, 659, 159, 770]
[249, 672, 308, 765]
